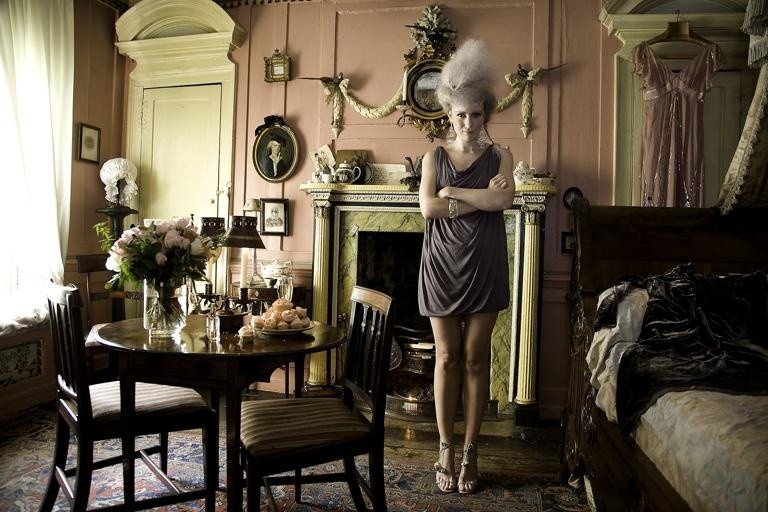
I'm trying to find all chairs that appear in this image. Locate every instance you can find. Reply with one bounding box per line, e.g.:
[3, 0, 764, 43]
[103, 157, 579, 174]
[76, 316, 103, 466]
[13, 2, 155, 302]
[240, 284, 392, 512]
[38, 281, 216, 512]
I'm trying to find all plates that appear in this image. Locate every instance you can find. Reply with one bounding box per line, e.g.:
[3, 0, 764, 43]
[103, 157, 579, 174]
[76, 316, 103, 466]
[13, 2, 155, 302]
[260, 325, 314, 335]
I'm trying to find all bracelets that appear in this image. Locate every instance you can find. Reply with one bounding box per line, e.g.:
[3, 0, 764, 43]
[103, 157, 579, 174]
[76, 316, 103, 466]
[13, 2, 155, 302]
[448, 198, 459, 219]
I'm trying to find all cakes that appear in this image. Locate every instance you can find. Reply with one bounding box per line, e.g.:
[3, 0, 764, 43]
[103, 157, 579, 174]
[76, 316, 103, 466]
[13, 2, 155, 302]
[238, 296, 310, 344]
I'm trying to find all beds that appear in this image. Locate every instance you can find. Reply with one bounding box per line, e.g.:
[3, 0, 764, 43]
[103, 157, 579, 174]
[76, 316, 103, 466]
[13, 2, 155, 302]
[565, 198, 768, 512]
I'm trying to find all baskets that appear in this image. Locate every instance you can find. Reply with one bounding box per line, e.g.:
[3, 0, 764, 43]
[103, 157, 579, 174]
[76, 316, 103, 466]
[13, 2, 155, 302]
[513, 168, 560, 192]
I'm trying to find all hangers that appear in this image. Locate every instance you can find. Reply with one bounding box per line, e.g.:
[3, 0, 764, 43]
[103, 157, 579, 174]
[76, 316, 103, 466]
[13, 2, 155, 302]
[645, 9, 710, 48]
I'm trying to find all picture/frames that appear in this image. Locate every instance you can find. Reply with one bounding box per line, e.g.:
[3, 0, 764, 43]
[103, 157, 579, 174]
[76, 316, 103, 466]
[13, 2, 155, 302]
[77, 122, 101, 165]
[253, 124, 299, 183]
[259, 197, 290, 236]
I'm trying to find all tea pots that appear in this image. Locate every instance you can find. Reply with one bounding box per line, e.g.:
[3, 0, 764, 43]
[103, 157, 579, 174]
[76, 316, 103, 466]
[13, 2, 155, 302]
[328, 160, 361, 185]
[259, 259, 293, 277]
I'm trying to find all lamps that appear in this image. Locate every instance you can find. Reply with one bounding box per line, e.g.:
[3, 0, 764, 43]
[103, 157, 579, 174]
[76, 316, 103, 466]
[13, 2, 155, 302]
[219, 215, 266, 249]
[241, 199, 262, 286]
[200, 217, 225, 237]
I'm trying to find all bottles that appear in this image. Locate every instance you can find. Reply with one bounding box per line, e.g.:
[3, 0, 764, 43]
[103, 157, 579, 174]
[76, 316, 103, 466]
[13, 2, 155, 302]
[206, 304, 221, 345]
[513, 161, 558, 187]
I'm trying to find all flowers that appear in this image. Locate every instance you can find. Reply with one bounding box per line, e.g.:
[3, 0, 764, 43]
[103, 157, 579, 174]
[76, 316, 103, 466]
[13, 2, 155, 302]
[104, 217, 215, 298]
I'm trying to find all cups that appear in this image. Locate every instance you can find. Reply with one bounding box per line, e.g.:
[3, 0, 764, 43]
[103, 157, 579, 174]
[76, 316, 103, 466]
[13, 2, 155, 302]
[264, 277, 277, 288]
[277, 277, 293, 301]
[322, 174, 333, 182]
[312, 170, 323, 183]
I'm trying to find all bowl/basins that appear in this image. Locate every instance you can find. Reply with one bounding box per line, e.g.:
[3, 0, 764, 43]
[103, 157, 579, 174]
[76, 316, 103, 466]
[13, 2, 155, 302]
[198, 309, 248, 324]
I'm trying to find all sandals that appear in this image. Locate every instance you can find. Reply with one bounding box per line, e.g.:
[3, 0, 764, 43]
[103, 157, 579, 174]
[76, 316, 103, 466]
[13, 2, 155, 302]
[459, 446, 479, 494]
[435, 442, 457, 493]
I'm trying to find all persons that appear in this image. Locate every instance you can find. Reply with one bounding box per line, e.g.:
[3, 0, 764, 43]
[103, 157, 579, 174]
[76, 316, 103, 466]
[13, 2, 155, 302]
[418, 37, 515, 495]
[265, 132, 287, 179]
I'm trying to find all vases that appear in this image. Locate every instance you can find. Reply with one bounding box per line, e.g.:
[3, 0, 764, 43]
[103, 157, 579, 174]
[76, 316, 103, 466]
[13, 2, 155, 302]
[144, 296, 186, 339]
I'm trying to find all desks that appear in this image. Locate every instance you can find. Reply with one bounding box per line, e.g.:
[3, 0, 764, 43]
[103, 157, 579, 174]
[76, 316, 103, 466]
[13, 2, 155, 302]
[239, 282, 306, 387]
